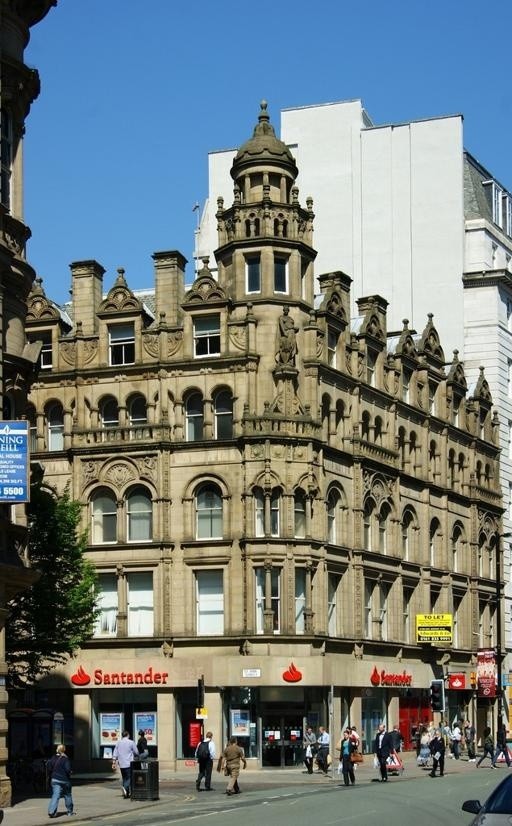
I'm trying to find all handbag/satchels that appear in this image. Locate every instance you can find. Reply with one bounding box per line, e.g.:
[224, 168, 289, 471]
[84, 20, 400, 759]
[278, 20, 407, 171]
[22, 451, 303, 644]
[144, 749, 149, 752]
[350, 749, 364, 762]
[224, 765, 230, 776]
[47, 777, 53, 798]
[310, 744, 317, 754]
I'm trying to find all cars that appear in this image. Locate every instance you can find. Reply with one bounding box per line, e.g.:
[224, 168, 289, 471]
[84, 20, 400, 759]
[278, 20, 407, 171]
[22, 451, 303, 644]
[461, 771, 512, 826]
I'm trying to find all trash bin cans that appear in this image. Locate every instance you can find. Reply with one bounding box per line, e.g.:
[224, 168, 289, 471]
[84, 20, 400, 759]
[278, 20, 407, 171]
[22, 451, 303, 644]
[129, 760, 159, 800]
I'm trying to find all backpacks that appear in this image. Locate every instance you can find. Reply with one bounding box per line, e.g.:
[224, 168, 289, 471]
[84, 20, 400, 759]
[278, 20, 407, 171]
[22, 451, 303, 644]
[196, 739, 212, 759]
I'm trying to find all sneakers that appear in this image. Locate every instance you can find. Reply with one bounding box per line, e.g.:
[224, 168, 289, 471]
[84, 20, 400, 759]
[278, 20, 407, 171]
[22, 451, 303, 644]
[121, 786, 129, 798]
[468, 758, 476, 763]
[428, 771, 435, 777]
[476, 763, 479, 768]
[490, 764, 501, 769]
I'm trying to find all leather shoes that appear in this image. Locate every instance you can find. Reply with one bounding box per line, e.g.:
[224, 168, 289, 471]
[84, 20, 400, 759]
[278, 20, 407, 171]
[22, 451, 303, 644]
[226, 790, 232, 796]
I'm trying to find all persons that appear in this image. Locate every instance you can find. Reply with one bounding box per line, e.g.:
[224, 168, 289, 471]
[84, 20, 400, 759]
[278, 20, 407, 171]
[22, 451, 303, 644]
[195, 732, 216, 790]
[340, 718, 476, 786]
[476, 727, 501, 768]
[279, 305, 299, 366]
[316, 726, 330, 776]
[112, 731, 140, 797]
[137, 730, 149, 759]
[302, 726, 317, 774]
[48, 744, 77, 817]
[490, 724, 512, 769]
[223, 736, 246, 795]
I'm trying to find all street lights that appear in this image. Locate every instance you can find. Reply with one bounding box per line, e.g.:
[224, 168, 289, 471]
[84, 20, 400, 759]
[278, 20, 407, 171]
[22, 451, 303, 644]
[493, 531, 511, 748]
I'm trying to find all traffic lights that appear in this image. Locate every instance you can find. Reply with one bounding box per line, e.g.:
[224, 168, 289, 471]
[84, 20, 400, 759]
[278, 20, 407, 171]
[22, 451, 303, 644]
[429, 679, 445, 712]
